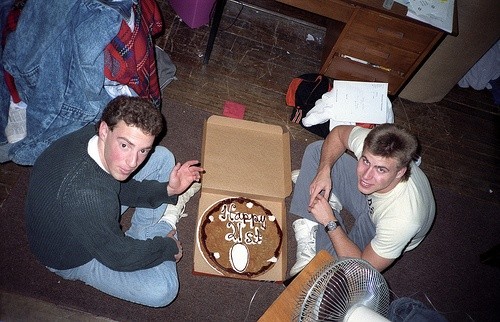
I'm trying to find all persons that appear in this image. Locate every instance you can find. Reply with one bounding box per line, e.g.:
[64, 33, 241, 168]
[25, 95, 207, 307]
[289, 124, 437, 277]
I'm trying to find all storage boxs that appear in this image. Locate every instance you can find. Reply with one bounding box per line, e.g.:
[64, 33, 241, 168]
[189, 112, 294, 284]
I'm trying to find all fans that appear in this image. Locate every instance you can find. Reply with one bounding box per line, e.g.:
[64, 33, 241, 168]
[291, 254, 394, 322]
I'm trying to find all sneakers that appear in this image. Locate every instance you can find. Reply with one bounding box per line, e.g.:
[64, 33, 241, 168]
[288, 217, 321, 276]
[164, 183, 201, 213]
[291, 170, 341, 210]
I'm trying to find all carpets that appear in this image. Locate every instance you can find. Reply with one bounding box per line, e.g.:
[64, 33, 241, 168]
[0, 96, 500, 321]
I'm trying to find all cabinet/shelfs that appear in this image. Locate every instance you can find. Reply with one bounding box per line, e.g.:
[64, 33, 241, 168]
[203, 0, 459, 96]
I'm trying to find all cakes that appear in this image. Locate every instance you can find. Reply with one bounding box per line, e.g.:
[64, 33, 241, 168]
[196, 197, 283, 279]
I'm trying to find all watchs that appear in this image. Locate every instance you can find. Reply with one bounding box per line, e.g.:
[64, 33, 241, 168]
[324, 221, 341, 233]
